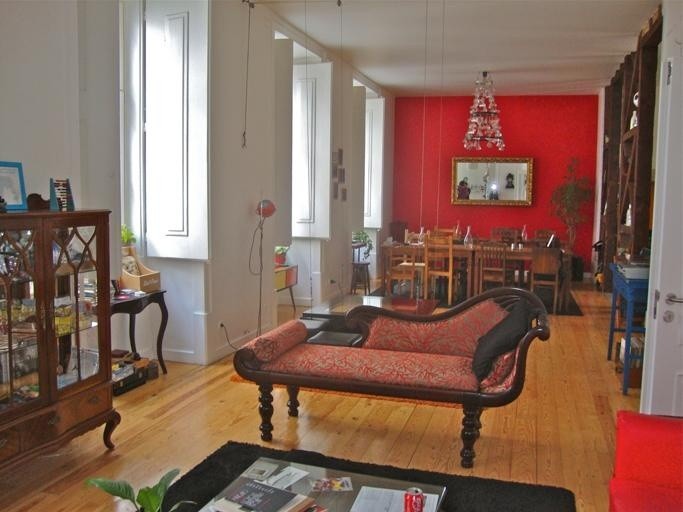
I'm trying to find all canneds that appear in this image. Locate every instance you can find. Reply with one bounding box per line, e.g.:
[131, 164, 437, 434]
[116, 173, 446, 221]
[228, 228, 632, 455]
[404, 487, 423, 512]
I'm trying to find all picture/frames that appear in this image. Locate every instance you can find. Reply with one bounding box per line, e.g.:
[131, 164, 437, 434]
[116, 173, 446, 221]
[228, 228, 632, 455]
[451, 157, 534, 206]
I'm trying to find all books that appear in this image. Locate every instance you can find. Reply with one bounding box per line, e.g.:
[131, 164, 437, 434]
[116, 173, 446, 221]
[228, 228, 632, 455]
[618, 335, 644, 369]
[76, 278, 117, 304]
[616, 254, 650, 279]
[211, 477, 329, 512]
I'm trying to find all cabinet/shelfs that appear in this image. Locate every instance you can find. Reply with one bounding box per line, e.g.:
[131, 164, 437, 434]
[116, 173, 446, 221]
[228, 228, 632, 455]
[0, 208, 119, 476]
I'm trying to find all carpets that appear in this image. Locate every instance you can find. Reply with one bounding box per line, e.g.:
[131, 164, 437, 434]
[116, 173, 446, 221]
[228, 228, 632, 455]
[371, 279, 583, 316]
[133, 440, 577, 512]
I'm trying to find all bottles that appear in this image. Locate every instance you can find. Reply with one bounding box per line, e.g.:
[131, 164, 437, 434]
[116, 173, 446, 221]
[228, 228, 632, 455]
[522, 224, 529, 241]
[419, 227, 425, 243]
[464, 226, 473, 248]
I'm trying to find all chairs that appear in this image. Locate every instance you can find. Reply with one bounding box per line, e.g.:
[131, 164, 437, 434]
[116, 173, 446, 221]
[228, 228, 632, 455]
[380, 222, 572, 313]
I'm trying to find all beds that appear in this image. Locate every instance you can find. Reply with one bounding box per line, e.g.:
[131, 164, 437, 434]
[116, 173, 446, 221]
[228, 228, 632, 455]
[233, 288, 549, 469]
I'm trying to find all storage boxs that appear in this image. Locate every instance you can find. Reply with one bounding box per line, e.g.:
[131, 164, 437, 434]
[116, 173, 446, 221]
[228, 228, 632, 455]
[616, 341, 642, 390]
[275, 265, 298, 285]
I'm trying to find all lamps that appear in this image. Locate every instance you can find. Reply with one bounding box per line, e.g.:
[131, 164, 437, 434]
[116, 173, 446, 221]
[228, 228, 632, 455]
[249, 199, 276, 339]
[462, 72, 506, 151]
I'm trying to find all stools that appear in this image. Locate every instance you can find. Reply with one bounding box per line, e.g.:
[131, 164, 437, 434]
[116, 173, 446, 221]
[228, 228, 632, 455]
[350, 263, 371, 295]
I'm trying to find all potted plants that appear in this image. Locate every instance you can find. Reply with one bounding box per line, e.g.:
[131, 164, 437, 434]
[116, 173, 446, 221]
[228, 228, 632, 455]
[352, 231, 373, 259]
[275, 247, 288, 263]
[551, 160, 587, 280]
[121, 224, 136, 256]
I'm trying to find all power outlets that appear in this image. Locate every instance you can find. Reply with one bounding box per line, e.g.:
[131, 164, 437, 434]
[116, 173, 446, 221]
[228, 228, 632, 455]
[218, 319, 226, 329]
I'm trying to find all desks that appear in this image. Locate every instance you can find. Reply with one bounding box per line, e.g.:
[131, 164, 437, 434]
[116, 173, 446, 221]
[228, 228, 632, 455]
[276, 286, 296, 313]
[110, 288, 170, 377]
[300, 294, 440, 318]
[607, 264, 650, 396]
